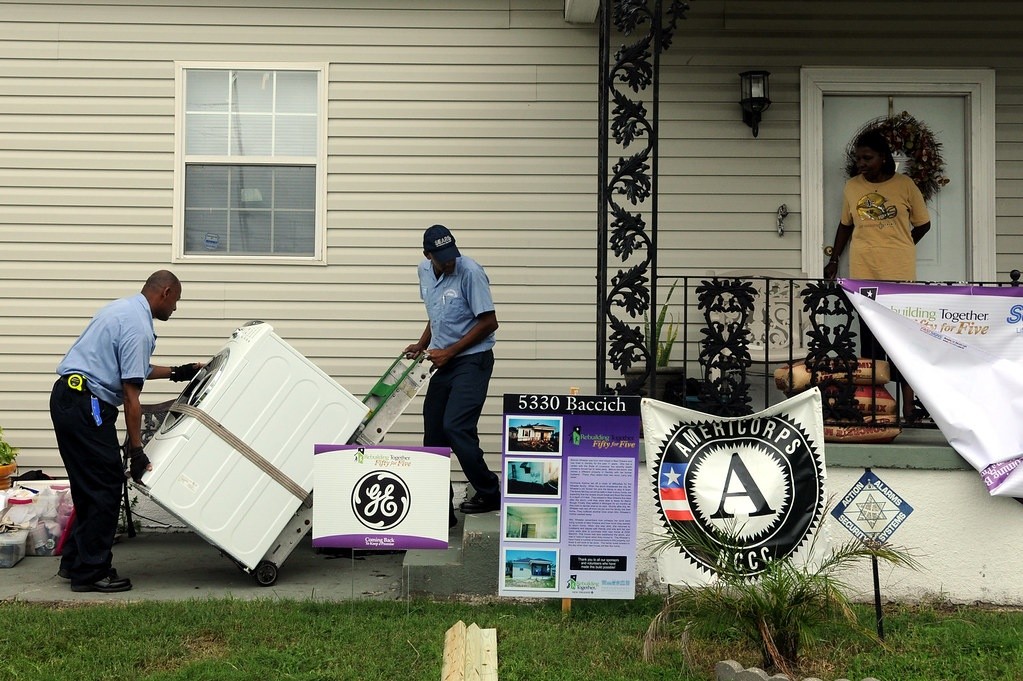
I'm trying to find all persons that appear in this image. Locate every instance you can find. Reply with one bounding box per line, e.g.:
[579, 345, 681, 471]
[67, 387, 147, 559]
[404, 225, 500, 530]
[50, 269, 207, 594]
[824, 132, 932, 422]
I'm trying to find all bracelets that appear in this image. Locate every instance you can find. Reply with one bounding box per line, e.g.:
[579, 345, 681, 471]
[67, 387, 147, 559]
[829, 261, 838, 265]
[169, 366, 175, 381]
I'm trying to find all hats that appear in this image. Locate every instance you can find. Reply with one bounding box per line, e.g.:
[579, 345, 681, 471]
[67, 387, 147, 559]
[424, 225, 461, 262]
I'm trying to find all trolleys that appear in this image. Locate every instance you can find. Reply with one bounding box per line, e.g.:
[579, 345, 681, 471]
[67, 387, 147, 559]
[253, 348, 438, 586]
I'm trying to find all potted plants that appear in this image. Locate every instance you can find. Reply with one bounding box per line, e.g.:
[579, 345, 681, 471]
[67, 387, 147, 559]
[0, 426, 21, 490]
[623, 279, 684, 408]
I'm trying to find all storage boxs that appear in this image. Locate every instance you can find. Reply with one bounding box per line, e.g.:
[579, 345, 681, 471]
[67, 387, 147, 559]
[0, 530, 29, 568]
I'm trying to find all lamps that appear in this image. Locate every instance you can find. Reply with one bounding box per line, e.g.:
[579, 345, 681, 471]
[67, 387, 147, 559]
[738, 70, 772, 138]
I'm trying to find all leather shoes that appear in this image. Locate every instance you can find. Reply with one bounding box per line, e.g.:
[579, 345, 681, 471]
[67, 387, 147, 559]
[449, 508, 457, 526]
[58, 568, 117, 580]
[460, 494, 502, 514]
[71, 575, 132, 592]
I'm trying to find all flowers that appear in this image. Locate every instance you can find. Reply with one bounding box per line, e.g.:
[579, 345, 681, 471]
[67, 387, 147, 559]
[844, 111, 951, 202]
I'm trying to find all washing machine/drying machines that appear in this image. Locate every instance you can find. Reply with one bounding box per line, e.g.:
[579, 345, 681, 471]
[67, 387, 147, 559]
[130, 321, 370, 573]
